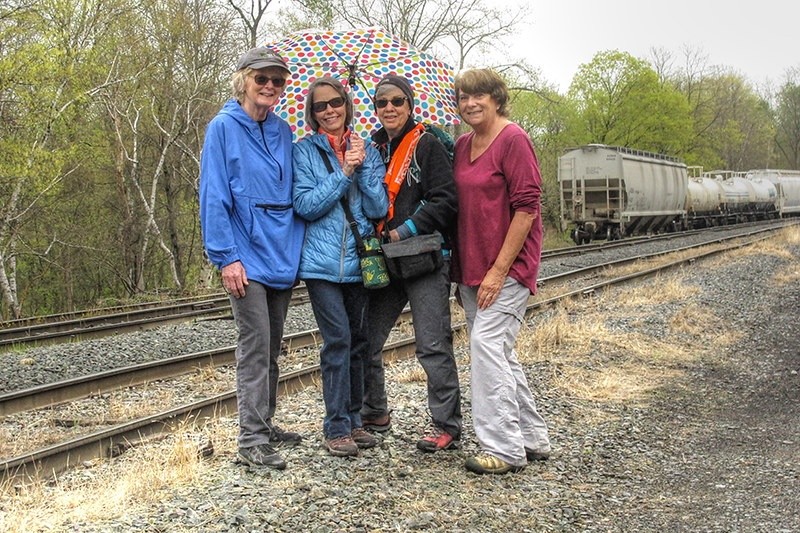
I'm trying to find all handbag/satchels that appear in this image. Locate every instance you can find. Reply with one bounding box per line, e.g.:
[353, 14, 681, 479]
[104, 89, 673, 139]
[381, 231, 444, 283]
[358, 236, 390, 289]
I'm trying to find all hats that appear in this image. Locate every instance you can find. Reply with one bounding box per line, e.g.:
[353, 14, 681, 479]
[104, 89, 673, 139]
[372, 73, 415, 114]
[236, 46, 292, 75]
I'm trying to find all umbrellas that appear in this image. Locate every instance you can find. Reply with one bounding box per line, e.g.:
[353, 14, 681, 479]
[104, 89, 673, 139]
[261, 26, 461, 143]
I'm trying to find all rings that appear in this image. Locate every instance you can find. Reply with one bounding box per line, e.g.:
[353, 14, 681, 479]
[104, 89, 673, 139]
[355, 142, 358, 146]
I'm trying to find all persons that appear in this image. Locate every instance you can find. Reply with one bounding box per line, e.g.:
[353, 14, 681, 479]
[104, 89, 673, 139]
[453, 70, 550, 474]
[198, 48, 306, 470]
[292, 77, 390, 457]
[360, 75, 462, 454]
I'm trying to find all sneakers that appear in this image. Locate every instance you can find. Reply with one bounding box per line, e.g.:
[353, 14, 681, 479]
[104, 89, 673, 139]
[360, 409, 393, 432]
[236, 443, 285, 471]
[269, 425, 301, 448]
[418, 425, 453, 452]
[322, 435, 358, 456]
[352, 427, 376, 448]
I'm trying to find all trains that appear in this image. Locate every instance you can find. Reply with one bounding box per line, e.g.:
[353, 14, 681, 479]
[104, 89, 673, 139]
[558, 143, 800, 245]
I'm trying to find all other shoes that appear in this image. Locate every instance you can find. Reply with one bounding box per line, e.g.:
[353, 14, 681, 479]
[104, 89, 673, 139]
[525, 451, 551, 460]
[466, 451, 527, 475]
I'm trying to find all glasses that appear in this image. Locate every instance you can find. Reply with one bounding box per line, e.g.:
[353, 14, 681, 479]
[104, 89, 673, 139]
[311, 96, 345, 112]
[255, 74, 286, 87]
[374, 98, 409, 108]
